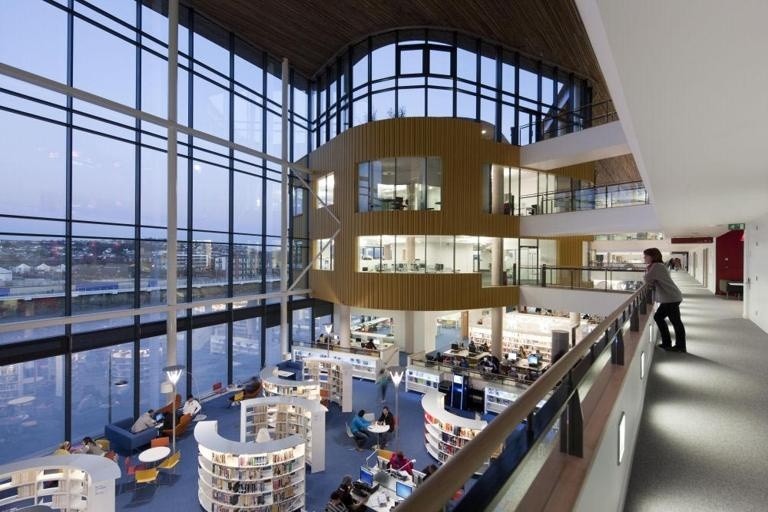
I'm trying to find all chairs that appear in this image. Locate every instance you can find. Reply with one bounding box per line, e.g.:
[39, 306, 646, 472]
[345, 422, 358, 451]
[100, 394, 192, 492]
[363, 413, 376, 422]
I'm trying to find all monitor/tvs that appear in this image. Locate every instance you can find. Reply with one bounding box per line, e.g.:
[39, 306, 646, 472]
[508, 353, 517, 361]
[528, 355, 538, 365]
[359, 466, 373, 487]
[395, 482, 413, 500]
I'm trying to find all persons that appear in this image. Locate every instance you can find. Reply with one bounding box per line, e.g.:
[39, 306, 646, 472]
[52, 440, 73, 455]
[319, 333, 325, 342]
[324, 490, 350, 512]
[642, 247, 688, 353]
[668, 257, 682, 271]
[129, 408, 158, 434]
[337, 475, 368, 511]
[421, 464, 438, 482]
[377, 405, 395, 450]
[176, 393, 202, 424]
[366, 339, 377, 350]
[351, 409, 372, 451]
[389, 450, 413, 475]
[360, 343, 367, 349]
[375, 367, 389, 402]
[433, 340, 543, 382]
[228, 375, 261, 401]
[80, 436, 104, 457]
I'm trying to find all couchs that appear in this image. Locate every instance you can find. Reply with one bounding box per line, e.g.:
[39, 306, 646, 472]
[719, 279, 743, 301]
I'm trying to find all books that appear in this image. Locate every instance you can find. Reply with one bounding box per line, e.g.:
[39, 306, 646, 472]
[9, 467, 89, 512]
[421, 409, 482, 467]
[243, 380, 312, 444]
[301, 359, 343, 406]
[194, 442, 304, 511]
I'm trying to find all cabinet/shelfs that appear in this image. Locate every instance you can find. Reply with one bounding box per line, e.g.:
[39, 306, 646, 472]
[404, 366, 443, 393]
[421, 390, 502, 477]
[484, 383, 547, 421]
[302, 357, 353, 412]
[0, 453, 122, 512]
[193, 420, 306, 512]
[290, 345, 381, 382]
[239, 396, 329, 474]
[260, 367, 320, 399]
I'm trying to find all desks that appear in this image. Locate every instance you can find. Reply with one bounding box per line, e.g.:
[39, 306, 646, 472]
[348, 459, 424, 512]
[444, 348, 549, 377]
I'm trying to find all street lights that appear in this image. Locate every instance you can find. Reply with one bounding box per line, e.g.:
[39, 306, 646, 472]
[163, 365, 185, 454]
[322, 324, 333, 357]
[386, 366, 407, 439]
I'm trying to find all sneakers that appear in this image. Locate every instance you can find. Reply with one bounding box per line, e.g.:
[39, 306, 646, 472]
[658, 343, 686, 353]
